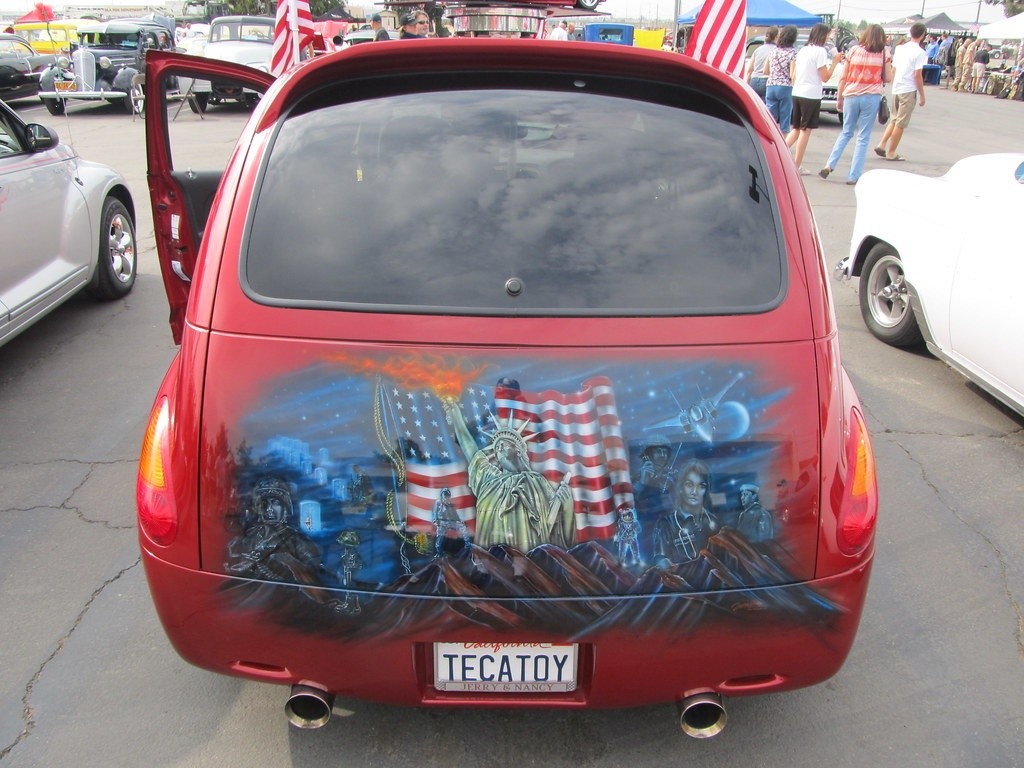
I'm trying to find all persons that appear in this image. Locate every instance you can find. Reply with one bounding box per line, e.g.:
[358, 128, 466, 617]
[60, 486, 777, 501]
[352, 24, 357, 31]
[746, 26, 780, 104]
[786, 23, 843, 175]
[301, 41, 314, 59]
[546, 20, 576, 41]
[175, 23, 194, 41]
[925, 32, 992, 94]
[897, 38, 905, 45]
[763, 25, 797, 138]
[818, 24, 892, 184]
[874, 23, 926, 161]
[333, 35, 348, 52]
[370, 10, 429, 42]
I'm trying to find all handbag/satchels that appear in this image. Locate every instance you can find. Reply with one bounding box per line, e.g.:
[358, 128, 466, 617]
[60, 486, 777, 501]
[878, 96, 890, 124]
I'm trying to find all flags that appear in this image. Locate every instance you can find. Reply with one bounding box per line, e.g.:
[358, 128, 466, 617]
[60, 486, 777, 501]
[685, 0, 746, 79]
[271, 0, 315, 77]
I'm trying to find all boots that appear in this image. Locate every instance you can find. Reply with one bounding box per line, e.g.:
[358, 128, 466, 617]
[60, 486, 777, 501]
[952, 84, 958, 91]
[958, 84, 968, 93]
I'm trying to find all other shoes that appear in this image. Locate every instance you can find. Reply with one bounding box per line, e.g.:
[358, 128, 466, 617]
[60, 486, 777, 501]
[971, 91, 978, 94]
[818, 169, 829, 178]
[847, 179, 855, 185]
[797, 166, 810, 176]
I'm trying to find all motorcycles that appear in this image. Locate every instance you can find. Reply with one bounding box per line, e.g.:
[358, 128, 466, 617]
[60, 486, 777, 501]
[582, 22, 634, 45]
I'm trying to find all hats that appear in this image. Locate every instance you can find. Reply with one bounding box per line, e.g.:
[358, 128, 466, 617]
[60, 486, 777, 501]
[371, 14, 381, 21]
[400, 15, 418, 25]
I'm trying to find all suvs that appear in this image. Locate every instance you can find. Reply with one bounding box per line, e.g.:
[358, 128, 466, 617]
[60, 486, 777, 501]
[38, 17, 178, 116]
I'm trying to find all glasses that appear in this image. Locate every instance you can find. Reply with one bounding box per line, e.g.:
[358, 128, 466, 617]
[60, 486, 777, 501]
[418, 19, 430, 24]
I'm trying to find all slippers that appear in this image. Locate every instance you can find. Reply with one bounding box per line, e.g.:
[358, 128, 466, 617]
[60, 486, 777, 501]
[875, 147, 886, 157]
[887, 153, 905, 161]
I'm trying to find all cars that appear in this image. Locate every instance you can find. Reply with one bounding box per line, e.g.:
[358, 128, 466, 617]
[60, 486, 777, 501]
[173, 0, 401, 114]
[832, 151, 1024, 420]
[0, 95, 139, 351]
[988, 44, 1002, 60]
[746, 35, 845, 124]
[143, 35, 879, 741]
[12, 18, 101, 54]
[0, 33, 71, 103]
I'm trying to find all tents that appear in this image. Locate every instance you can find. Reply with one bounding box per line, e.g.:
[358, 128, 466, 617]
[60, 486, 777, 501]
[312, 5, 366, 29]
[881, 11, 971, 36]
[676, 0, 822, 37]
[977, 11, 1024, 39]
